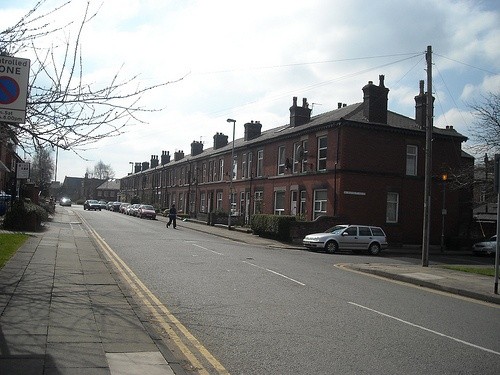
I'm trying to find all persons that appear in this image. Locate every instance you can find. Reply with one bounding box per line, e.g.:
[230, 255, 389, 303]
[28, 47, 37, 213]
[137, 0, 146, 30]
[167, 204, 177, 229]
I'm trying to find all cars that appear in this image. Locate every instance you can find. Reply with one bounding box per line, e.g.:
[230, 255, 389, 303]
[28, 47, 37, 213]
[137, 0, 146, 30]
[60, 196, 71, 206]
[128, 204, 142, 216]
[472, 234, 497, 257]
[138, 204, 156, 219]
[83, 199, 101, 211]
[98, 201, 131, 215]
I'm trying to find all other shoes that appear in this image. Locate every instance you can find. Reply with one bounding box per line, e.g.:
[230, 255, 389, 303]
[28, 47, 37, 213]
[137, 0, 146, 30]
[173, 226, 176, 229]
[166, 223, 168, 228]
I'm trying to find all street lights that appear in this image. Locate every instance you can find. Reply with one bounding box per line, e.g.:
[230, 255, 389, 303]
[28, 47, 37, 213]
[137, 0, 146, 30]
[226, 118, 236, 230]
[53, 137, 64, 209]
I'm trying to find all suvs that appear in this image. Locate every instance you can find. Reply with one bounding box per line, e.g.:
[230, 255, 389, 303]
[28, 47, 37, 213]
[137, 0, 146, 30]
[302, 224, 388, 256]
